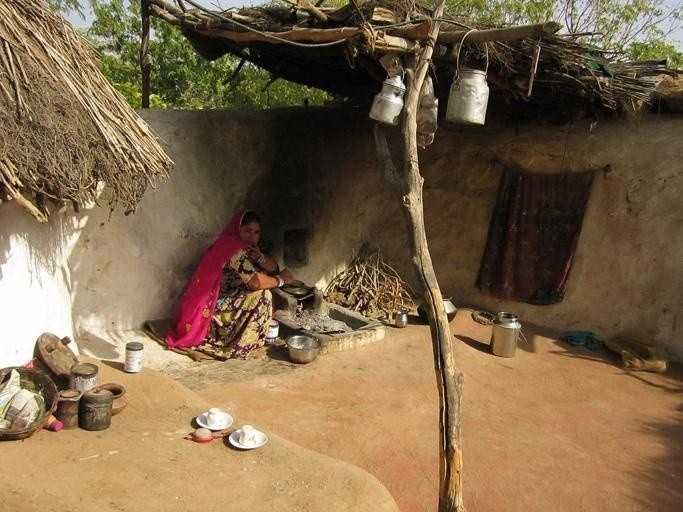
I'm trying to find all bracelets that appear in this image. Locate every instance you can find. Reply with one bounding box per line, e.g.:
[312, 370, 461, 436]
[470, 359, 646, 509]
[273, 275, 284, 287]
[256, 253, 266, 264]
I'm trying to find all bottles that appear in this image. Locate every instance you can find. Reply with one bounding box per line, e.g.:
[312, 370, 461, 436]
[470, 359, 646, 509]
[394, 311, 407, 328]
[44, 416, 63, 432]
[123, 342, 143, 372]
[489, 312, 521, 359]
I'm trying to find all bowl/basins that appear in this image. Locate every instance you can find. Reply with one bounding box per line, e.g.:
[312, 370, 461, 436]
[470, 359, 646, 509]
[285, 335, 322, 364]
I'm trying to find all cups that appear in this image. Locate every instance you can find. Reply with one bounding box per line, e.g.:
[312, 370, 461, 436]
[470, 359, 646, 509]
[238, 425, 254, 443]
[206, 407, 222, 425]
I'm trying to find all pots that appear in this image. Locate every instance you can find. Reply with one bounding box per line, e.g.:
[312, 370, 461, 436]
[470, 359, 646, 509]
[282, 281, 312, 295]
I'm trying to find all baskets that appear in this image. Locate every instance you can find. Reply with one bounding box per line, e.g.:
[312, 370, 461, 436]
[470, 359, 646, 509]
[0, 366, 60, 441]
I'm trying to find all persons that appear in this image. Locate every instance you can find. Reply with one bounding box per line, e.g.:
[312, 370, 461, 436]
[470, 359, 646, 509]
[165, 208, 293, 362]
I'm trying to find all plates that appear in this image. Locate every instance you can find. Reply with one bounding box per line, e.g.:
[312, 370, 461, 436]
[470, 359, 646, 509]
[195, 410, 233, 431]
[227, 428, 267, 450]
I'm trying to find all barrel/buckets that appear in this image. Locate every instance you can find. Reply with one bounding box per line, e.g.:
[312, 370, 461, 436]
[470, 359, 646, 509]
[367, 64, 408, 127]
[69, 362, 98, 392]
[445, 29, 490, 127]
[488, 310, 528, 358]
